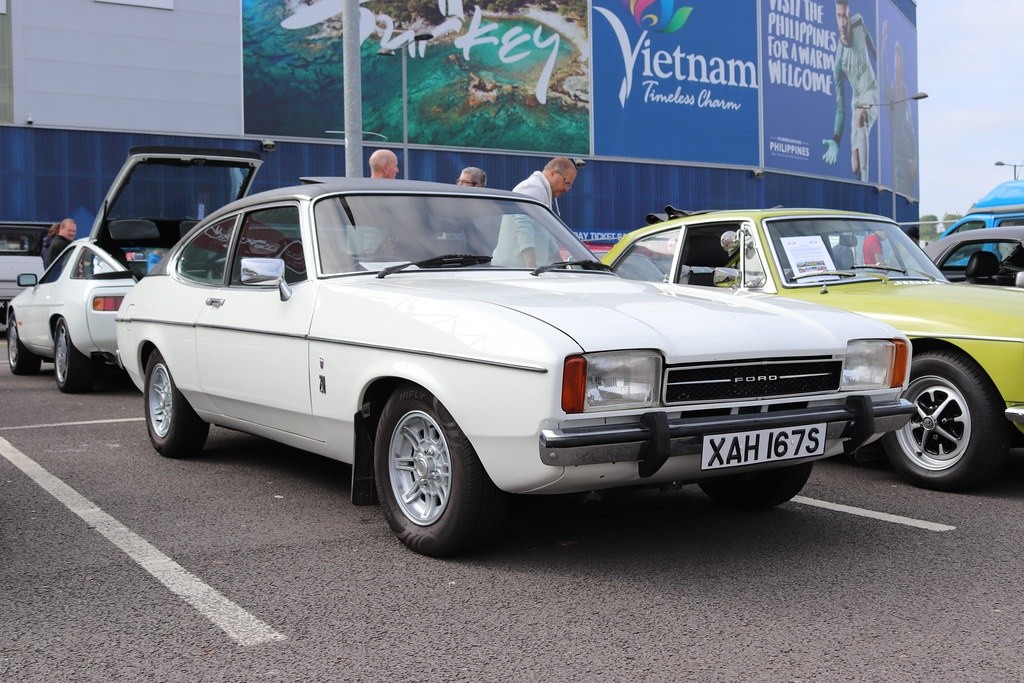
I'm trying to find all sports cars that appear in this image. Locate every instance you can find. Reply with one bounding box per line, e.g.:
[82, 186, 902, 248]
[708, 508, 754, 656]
[109, 176, 918, 561]
[597, 205, 1024, 488]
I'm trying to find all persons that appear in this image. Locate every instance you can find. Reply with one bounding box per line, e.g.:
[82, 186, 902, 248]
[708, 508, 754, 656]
[369, 149, 399, 178]
[456, 166, 487, 188]
[48, 219, 77, 266]
[490, 156, 576, 269]
[881, 19, 918, 198]
[863, 230, 888, 265]
[832, 232, 854, 270]
[41, 224, 60, 270]
[821, 0, 878, 182]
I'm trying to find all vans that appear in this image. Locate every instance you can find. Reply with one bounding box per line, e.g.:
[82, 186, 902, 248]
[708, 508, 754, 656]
[935, 179, 1024, 267]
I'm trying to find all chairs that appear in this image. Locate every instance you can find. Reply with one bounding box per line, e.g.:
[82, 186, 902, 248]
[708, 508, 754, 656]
[965, 251, 1000, 284]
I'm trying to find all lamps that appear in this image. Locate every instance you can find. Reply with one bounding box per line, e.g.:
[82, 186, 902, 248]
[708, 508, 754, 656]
[753, 169, 765, 178]
[572, 157, 586, 168]
[261, 139, 277, 151]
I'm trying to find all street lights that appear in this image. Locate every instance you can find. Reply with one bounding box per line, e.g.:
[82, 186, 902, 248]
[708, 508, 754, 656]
[400, 27, 437, 178]
[852, 91, 932, 229]
[995, 161, 1024, 182]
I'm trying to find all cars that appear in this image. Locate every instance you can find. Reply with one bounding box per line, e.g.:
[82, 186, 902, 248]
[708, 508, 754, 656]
[910, 224, 1024, 288]
[5, 150, 264, 393]
[0, 222, 58, 314]
[553, 246, 668, 288]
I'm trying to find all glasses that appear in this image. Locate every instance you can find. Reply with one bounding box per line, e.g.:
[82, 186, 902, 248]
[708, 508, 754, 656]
[456, 179, 480, 187]
[556, 170, 573, 189]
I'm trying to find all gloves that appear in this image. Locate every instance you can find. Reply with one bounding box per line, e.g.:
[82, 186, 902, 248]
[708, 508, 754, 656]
[822, 139, 839, 165]
